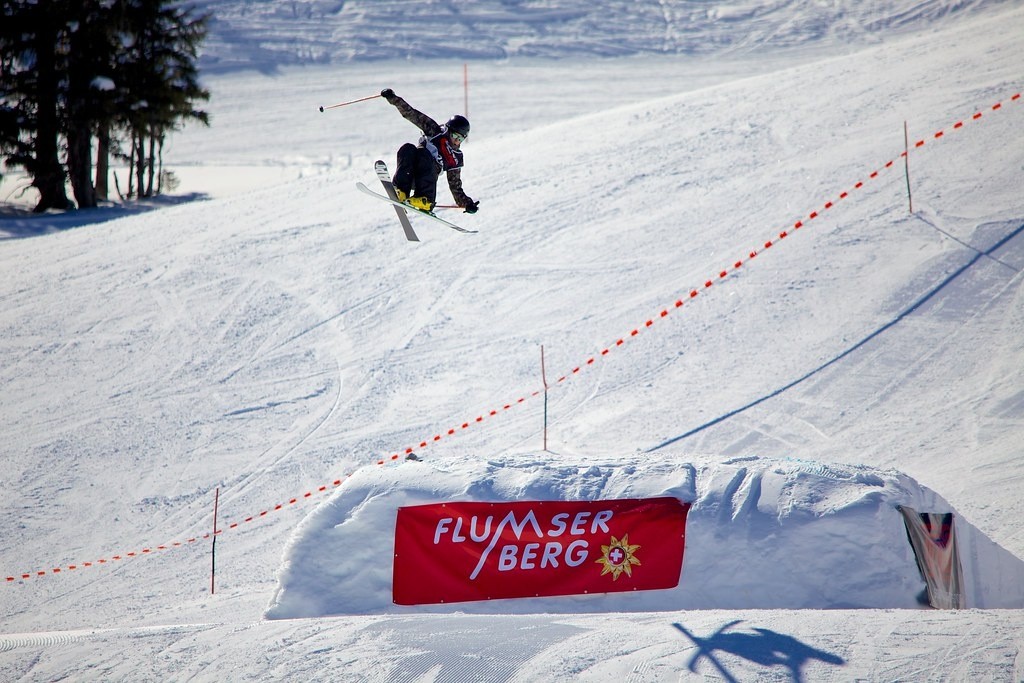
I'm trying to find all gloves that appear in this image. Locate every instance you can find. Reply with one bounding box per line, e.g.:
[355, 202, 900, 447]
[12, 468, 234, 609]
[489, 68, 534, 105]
[381, 88, 394, 99]
[465, 201, 479, 214]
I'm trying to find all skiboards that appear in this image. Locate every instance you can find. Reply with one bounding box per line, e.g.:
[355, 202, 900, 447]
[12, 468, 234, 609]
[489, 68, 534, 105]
[358, 160, 421, 241]
[355, 182, 479, 234]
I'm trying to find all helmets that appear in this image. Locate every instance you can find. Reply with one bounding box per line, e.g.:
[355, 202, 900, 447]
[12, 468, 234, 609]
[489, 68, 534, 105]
[445, 115, 470, 139]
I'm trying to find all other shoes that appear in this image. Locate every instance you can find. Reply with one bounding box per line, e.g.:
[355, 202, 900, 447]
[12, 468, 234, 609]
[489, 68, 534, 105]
[393, 186, 406, 203]
[402, 195, 434, 212]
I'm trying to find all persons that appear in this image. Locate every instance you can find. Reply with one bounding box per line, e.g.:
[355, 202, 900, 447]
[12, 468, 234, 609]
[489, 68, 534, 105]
[380, 88, 478, 214]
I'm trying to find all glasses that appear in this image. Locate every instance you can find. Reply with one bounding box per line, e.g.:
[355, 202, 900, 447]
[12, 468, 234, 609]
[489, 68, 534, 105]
[448, 128, 465, 143]
